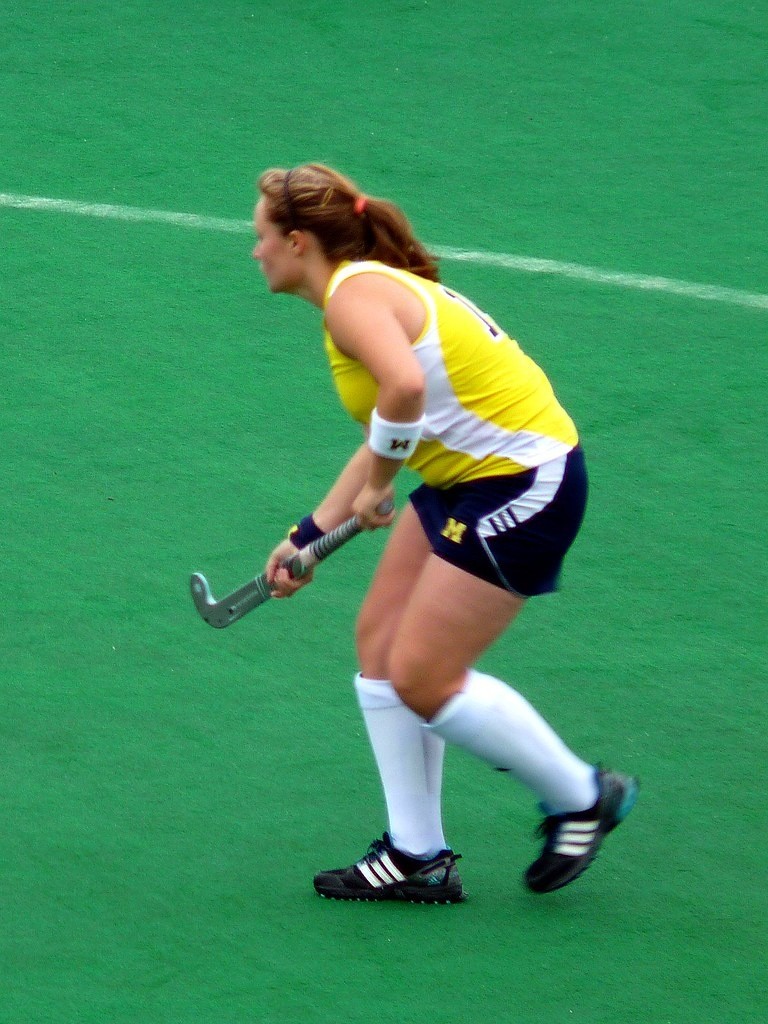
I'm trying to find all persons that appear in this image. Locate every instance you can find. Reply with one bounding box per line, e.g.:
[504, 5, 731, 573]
[253, 161, 641, 905]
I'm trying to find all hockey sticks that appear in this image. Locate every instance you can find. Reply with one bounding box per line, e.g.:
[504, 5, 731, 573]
[189, 497, 394, 628]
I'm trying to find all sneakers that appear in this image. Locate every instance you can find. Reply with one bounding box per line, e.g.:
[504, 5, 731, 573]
[314, 832, 468, 905]
[526, 762, 639, 894]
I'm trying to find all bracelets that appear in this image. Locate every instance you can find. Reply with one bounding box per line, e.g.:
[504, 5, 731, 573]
[288, 513, 325, 550]
